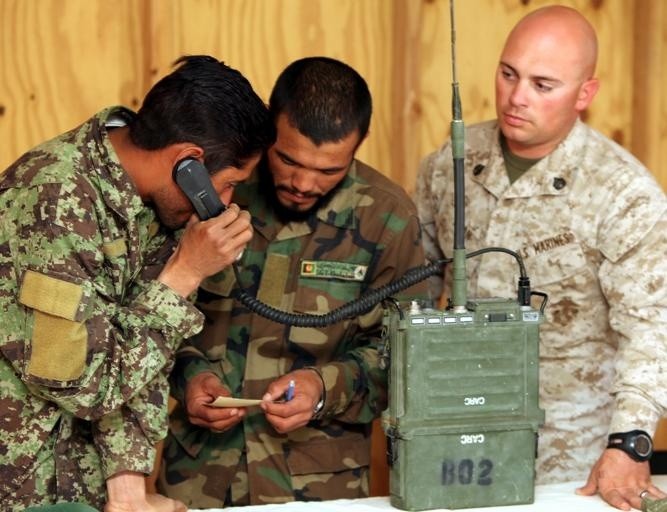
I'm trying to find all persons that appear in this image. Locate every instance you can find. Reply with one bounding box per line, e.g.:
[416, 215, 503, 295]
[404, 3, 667, 512]
[1, 52, 280, 512]
[143, 55, 431, 511]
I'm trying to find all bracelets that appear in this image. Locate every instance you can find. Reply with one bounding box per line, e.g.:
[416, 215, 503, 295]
[314, 390, 326, 414]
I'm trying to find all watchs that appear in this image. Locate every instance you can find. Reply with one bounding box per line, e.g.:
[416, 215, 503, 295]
[605, 428, 654, 463]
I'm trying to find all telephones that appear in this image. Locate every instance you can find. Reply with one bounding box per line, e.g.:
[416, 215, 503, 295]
[171, 156, 246, 263]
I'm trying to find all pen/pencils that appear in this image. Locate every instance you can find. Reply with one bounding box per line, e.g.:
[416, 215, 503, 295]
[286, 380, 294, 400]
